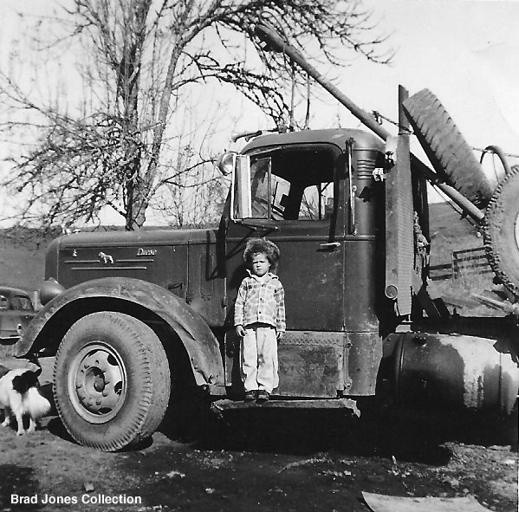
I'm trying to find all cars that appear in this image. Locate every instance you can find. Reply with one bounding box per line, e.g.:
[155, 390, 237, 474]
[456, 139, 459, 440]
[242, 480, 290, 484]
[1, 286, 36, 342]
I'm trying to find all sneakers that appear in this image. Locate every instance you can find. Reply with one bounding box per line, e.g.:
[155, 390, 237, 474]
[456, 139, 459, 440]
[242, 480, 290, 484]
[258, 391, 269, 400]
[246, 391, 257, 401]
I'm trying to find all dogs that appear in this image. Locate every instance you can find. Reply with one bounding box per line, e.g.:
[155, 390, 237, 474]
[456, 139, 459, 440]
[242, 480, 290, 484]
[0, 365, 51, 436]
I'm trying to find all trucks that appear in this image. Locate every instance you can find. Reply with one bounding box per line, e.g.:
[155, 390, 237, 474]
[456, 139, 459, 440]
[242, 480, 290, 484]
[12, 26, 519, 452]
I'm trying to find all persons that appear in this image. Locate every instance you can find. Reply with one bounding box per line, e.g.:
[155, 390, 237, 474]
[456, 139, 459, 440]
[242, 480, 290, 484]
[232, 236, 286, 402]
